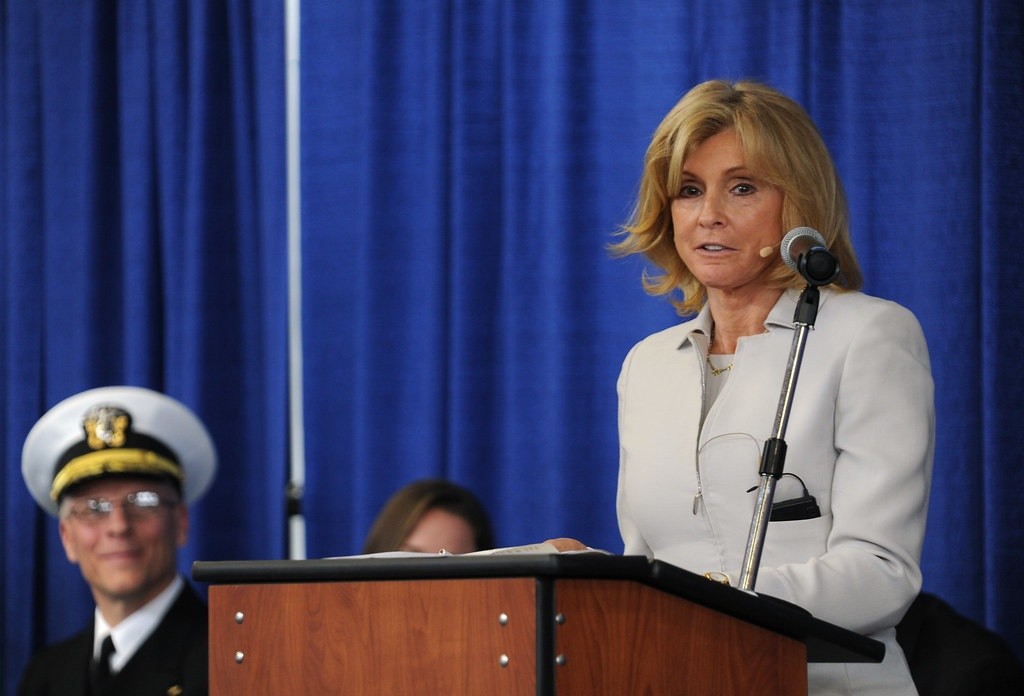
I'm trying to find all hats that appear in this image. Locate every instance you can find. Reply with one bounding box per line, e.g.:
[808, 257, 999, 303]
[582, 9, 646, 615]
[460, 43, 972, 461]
[19, 385, 218, 510]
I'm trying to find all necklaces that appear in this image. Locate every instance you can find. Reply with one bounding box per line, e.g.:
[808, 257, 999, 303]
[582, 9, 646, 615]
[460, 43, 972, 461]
[705, 329, 734, 376]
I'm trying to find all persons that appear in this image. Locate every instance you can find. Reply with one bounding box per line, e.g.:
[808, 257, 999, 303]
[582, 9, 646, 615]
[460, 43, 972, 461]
[14, 387, 219, 696]
[363, 479, 493, 554]
[545, 81, 936, 696]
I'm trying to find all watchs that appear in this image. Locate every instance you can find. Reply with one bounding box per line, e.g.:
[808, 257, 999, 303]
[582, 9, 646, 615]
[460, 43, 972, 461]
[704, 572, 730, 586]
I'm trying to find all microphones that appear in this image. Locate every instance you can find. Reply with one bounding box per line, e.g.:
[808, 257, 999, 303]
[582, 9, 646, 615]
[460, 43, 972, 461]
[780, 226, 840, 286]
[759, 241, 782, 258]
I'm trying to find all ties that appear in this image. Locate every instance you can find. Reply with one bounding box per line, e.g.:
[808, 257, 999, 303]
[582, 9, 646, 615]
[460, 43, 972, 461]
[89, 632, 120, 694]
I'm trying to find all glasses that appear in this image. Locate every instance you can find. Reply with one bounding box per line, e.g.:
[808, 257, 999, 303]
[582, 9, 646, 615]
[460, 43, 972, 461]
[62, 488, 183, 518]
